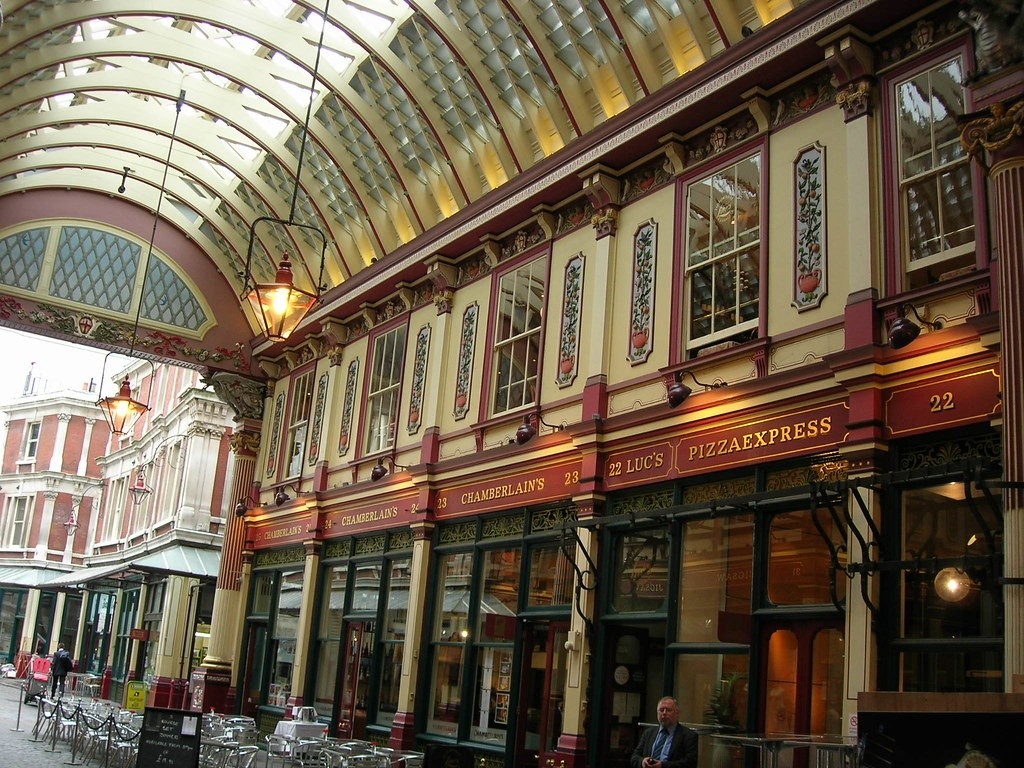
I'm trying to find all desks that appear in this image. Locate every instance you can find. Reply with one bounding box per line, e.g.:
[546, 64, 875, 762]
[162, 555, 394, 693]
[68, 673, 90, 694]
[277, 721, 328, 761]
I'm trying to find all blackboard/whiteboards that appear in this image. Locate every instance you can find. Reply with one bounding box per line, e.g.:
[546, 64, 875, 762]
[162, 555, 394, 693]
[135, 707, 203, 768]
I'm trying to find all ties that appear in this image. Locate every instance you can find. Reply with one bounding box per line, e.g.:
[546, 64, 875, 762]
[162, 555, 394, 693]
[652, 728, 668, 760]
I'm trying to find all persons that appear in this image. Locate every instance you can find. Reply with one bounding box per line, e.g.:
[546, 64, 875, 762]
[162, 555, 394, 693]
[51, 642, 69, 699]
[629, 695, 698, 768]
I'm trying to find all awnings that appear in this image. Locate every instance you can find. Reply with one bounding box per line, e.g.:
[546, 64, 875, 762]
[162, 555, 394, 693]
[35, 564, 146, 594]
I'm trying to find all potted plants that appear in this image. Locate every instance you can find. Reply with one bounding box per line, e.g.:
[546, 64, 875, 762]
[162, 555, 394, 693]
[700, 670, 749, 768]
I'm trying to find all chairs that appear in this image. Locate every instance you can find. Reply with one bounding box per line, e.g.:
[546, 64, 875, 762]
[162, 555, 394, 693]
[29, 671, 427, 768]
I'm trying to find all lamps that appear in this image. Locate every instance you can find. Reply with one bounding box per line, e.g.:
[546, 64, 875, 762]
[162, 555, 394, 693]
[237, 0, 343, 343]
[668, 369, 728, 408]
[370, 454, 414, 483]
[887, 299, 944, 349]
[62, 483, 107, 537]
[128, 432, 190, 505]
[235, 495, 269, 517]
[514, 410, 565, 446]
[274, 483, 311, 509]
[96, 86, 189, 438]
